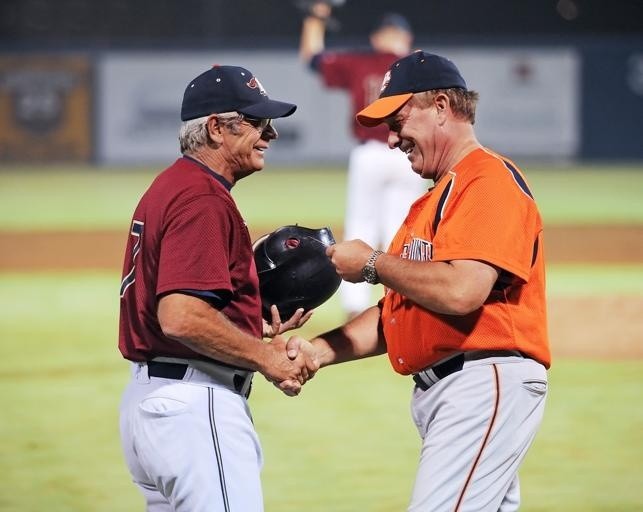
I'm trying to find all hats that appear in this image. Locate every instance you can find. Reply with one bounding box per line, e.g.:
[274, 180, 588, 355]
[181, 64, 296, 122]
[356, 49, 467, 128]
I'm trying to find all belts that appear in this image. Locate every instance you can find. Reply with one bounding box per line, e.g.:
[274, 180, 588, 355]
[144, 360, 253, 400]
[413, 349, 527, 392]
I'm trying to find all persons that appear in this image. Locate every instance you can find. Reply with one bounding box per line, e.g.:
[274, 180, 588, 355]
[298, 1, 427, 322]
[115, 64, 320, 512]
[276, 50, 552, 511]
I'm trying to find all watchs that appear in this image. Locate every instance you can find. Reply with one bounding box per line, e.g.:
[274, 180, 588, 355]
[360, 249, 382, 285]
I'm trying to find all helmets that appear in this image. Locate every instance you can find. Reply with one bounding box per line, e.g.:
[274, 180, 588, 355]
[251, 224, 342, 323]
